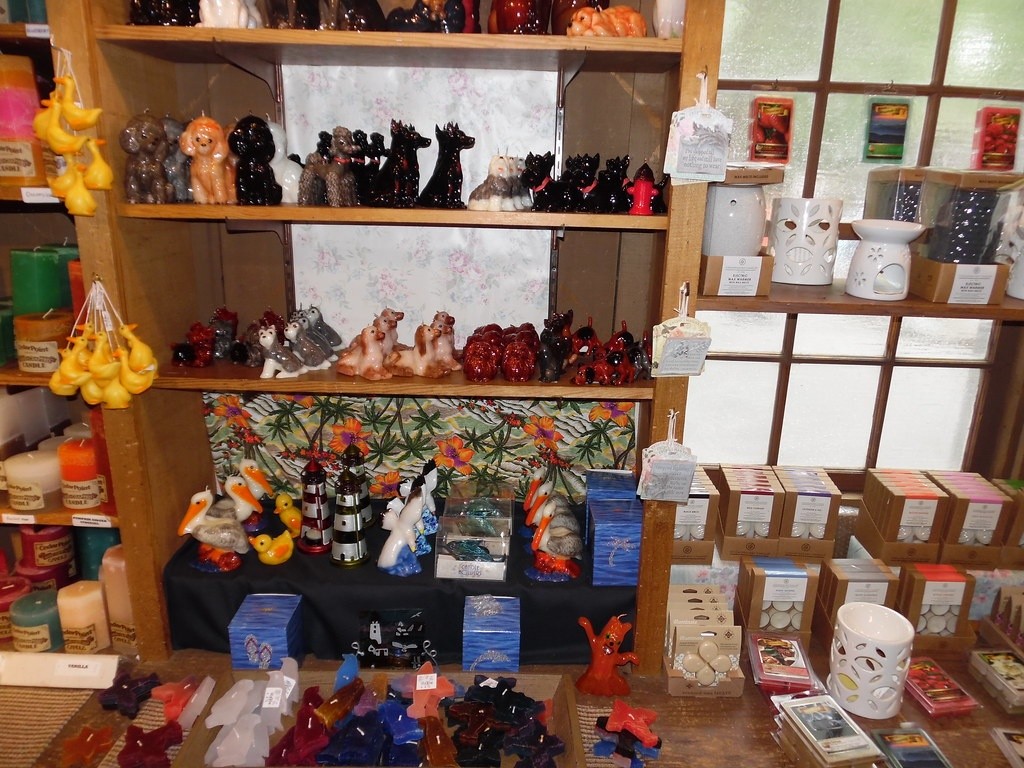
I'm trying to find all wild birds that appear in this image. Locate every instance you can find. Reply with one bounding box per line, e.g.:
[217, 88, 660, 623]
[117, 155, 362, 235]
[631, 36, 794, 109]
[376, 458, 438, 569]
[177, 459, 273, 571]
[523, 467, 584, 580]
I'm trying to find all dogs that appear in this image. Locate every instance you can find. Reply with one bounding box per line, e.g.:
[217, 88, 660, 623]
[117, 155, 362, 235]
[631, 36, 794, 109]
[467, 147, 668, 215]
[125, 0, 649, 40]
[119, 111, 476, 211]
[169, 306, 654, 388]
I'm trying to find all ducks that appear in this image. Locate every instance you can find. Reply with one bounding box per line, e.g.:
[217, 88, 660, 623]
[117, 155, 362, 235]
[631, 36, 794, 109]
[274, 493, 302, 539]
[248, 530, 294, 565]
[47, 323, 160, 410]
[31, 75, 115, 217]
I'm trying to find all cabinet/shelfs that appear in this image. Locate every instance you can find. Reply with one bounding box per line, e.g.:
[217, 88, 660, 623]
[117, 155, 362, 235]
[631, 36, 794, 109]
[0, 0, 1024, 692]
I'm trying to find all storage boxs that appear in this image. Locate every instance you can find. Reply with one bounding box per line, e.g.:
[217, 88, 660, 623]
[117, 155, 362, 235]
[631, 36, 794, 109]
[584, 470, 637, 507]
[226, 592, 304, 668]
[700, 249, 774, 297]
[911, 254, 1013, 305]
[586, 501, 640, 588]
[662, 467, 1024, 768]
[436, 517, 513, 582]
[171, 670, 586, 768]
[461, 595, 522, 677]
